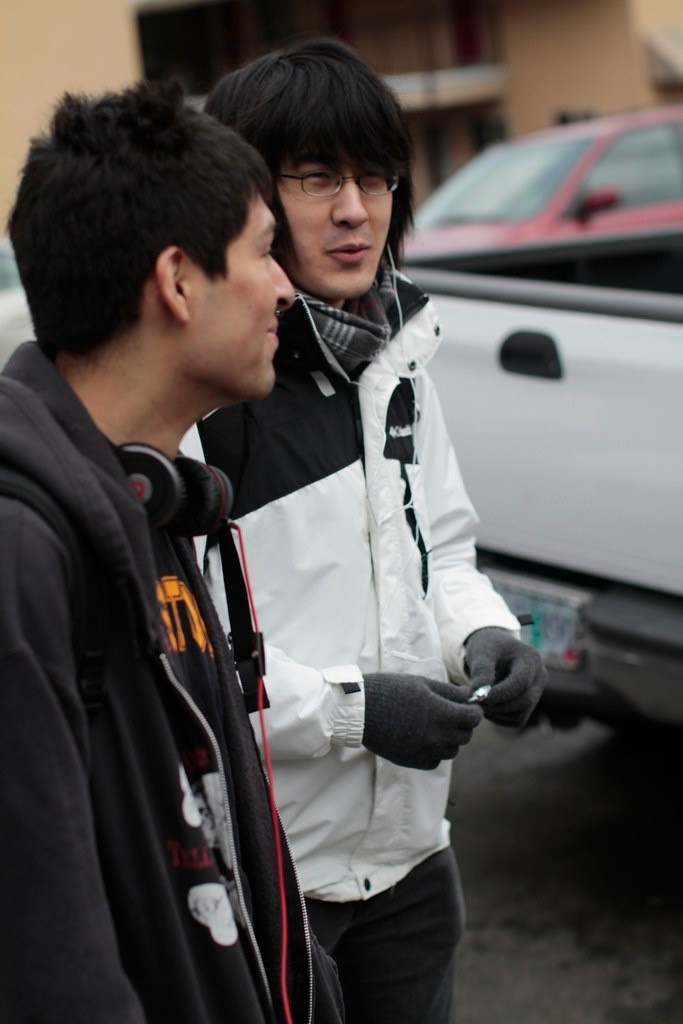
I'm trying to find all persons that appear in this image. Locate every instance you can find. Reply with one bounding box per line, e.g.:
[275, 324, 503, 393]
[0, 79, 346, 1023]
[179, 40, 551, 1024]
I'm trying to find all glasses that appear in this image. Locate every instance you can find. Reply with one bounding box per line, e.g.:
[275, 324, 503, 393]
[280, 165, 399, 195]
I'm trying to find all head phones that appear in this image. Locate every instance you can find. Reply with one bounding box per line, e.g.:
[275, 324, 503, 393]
[106, 439, 235, 536]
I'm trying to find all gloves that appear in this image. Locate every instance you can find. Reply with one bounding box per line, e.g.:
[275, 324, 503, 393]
[466, 627, 549, 729]
[362, 673, 482, 771]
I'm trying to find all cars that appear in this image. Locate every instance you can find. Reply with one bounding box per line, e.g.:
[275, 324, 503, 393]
[393, 106, 682, 264]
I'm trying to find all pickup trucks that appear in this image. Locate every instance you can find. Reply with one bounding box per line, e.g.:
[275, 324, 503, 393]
[394, 224, 683, 803]
